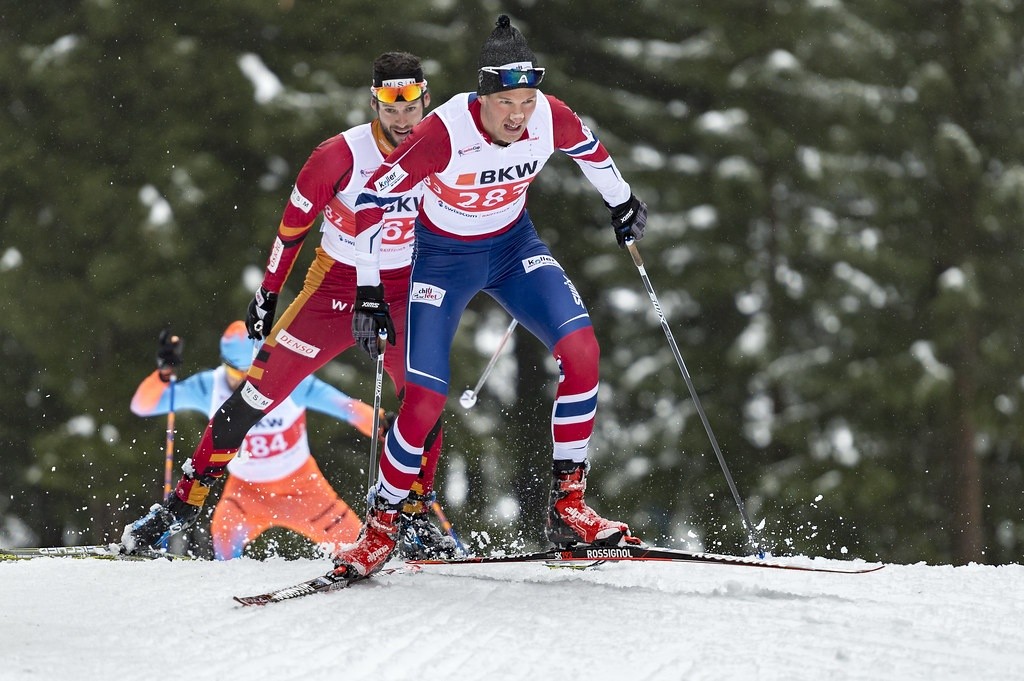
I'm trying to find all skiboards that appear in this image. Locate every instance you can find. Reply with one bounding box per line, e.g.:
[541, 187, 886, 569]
[0, 547, 606, 575]
[232, 528, 889, 609]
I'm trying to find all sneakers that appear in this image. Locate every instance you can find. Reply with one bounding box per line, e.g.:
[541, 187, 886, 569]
[395, 511, 457, 559]
[124, 492, 203, 550]
[332, 511, 399, 577]
[547, 478, 630, 545]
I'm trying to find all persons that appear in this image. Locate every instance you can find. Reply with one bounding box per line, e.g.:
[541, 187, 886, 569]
[120, 49, 457, 561]
[333, 29, 648, 579]
[128, 318, 399, 561]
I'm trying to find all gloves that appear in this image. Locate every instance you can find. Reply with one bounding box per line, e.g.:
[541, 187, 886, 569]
[604, 190, 648, 249]
[245, 285, 278, 340]
[352, 283, 397, 360]
[159, 331, 185, 372]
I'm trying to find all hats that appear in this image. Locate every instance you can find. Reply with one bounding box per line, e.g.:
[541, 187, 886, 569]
[476, 15, 538, 95]
[221, 321, 266, 369]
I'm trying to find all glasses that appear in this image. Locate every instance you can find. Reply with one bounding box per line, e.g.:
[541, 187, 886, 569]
[481, 66, 546, 86]
[371, 79, 427, 103]
